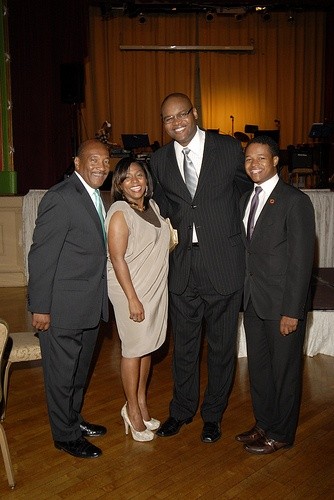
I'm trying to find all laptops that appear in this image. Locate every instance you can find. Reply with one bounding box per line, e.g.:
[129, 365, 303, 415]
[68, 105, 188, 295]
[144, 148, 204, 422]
[120, 133, 154, 152]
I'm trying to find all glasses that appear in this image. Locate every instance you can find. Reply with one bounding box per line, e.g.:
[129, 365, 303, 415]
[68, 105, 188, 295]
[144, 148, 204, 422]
[163, 107, 193, 123]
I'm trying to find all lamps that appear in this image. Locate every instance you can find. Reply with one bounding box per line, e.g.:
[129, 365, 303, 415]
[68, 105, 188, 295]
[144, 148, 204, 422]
[130, 6, 271, 23]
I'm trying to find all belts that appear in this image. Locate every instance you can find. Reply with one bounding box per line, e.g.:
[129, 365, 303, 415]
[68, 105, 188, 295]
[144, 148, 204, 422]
[192, 243, 198, 247]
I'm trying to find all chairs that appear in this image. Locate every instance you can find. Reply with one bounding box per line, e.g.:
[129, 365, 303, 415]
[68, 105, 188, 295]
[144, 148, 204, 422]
[4, 332, 41, 409]
[290, 150, 317, 189]
[0, 319, 17, 488]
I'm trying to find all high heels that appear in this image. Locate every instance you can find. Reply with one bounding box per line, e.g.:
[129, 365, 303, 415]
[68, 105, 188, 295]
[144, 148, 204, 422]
[142, 417, 160, 430]
[121, 401, 154, 442]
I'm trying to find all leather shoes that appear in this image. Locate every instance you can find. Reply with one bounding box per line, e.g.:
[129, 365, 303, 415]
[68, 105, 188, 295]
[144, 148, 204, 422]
[235, 428, 263, 442]
[79, 419, 106, 437]
[156, 415, 193, 437]
[243, 434, 291, 454]
[201, 422, 222, 442]
[53, 436, 102, 459]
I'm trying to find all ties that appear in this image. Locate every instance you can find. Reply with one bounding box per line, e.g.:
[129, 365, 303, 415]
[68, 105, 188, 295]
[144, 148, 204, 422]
[247, 187, 262, 242]
[182, 148, 199, 201]
[93, 190, 106, 242]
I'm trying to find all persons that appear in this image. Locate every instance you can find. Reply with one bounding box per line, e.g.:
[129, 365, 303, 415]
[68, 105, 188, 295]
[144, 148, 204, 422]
[147, 93, 254, 443]
[28, 139, 111, 458]
[104, 157, 175, 442]
[236, 136, 315, 454]
